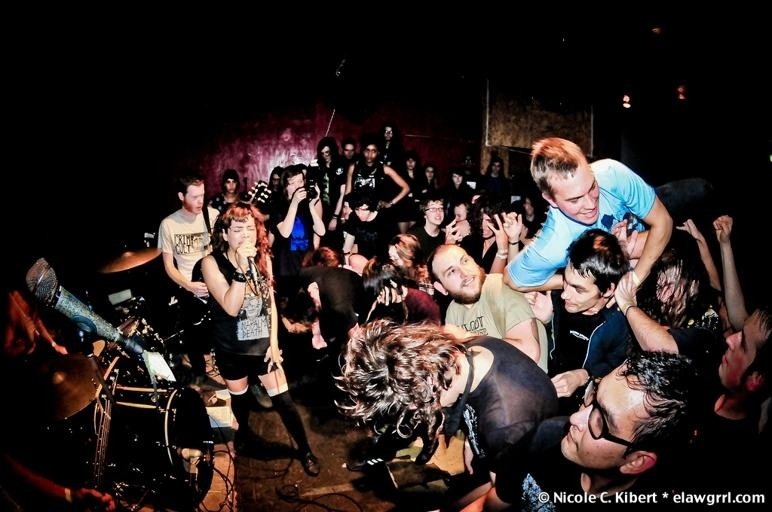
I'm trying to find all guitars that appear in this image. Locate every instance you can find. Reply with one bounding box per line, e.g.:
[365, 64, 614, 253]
[171, 181, 272, 330]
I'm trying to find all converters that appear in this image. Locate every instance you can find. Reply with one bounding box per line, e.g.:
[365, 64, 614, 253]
[276, 483, 297, 503]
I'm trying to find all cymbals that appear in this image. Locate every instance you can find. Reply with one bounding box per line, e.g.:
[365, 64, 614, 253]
[30, 354, 103, 419]
[99, 248, 163, 276]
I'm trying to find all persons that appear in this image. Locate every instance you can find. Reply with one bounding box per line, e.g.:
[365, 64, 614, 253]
[0, 285, 71, 370]
[0, 451, 117, 512]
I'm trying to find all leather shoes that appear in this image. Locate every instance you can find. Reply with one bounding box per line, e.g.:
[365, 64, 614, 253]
[249, 384, 273, 409]
[300, 448, 320, 476]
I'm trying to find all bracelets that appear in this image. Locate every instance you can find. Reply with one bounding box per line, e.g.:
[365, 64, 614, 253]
[50, 341, 57, 347]
[65, 487, 72, 501]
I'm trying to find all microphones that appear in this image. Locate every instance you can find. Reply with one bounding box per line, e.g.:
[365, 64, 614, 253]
[247, 256, 260, 295]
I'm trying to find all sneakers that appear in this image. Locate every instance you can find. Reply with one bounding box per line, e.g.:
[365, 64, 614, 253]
[346, 450, 386, 471]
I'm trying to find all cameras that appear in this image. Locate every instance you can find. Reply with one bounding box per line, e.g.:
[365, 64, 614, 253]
[303, 179, 318, 199]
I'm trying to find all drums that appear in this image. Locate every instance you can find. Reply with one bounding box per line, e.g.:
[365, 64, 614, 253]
[113, 381, 213, 512]
[106, 316, 165, 385]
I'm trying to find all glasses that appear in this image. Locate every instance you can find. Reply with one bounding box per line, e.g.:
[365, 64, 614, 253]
[424, 207, 445, 213]
[581, 375, 636, 450]
